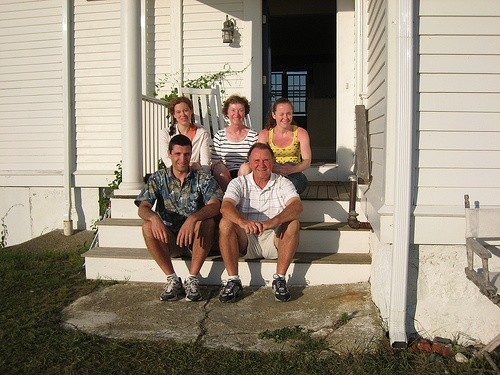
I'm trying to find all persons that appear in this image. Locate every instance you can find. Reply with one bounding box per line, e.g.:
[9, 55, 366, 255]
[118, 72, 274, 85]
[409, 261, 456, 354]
[258, 98, 311, 194]
[219, 142, 303, 302]
[134, 134, 224, 302]
[160, 96, 211, 174]
[210, 95, 260, 192]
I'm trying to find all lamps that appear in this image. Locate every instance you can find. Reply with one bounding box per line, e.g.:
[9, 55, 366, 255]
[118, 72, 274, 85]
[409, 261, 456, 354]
[221, 14, 237, 43]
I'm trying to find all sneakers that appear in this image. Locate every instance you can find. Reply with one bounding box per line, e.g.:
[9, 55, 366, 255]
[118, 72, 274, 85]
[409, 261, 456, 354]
[219, 279, 243, 303]
[184, 276, 202, 301]
[272, 274, 291, 301]
[159, 276, 185, 301]
